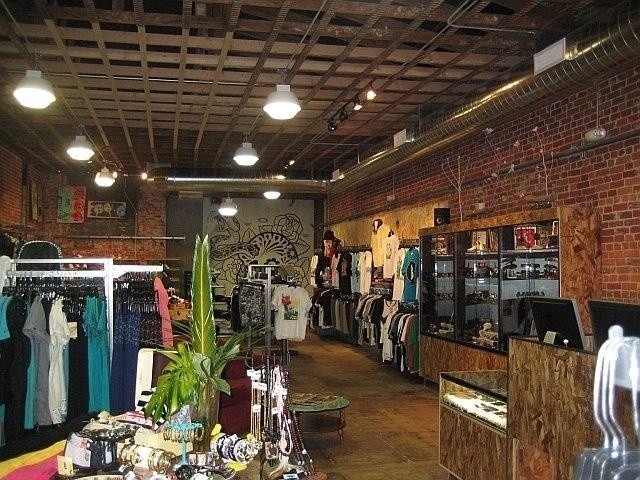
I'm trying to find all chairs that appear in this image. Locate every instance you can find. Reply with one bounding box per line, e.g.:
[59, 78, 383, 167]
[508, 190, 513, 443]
[153, 345, 282, 436]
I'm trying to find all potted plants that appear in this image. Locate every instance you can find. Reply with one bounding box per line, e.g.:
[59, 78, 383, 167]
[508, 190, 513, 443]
[143, 231, 275, 454]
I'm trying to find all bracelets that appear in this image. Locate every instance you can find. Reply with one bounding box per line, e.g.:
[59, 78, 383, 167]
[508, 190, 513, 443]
[118, 422, 251, 480]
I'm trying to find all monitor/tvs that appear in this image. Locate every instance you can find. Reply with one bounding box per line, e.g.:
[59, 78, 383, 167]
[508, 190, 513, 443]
[528, 296, 587, 350]
[588, 300, 640, 350]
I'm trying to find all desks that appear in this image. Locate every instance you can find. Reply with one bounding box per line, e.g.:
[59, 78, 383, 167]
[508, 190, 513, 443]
[288, 392, 350, 443]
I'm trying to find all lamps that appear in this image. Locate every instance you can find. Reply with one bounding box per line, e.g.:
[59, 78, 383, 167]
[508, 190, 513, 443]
[261, 73, 301, 119]
[217, 134, 283, 217]
[12, 50, 58, 109]
[66, 131, 147, 189]
[327, 82, 377, 131]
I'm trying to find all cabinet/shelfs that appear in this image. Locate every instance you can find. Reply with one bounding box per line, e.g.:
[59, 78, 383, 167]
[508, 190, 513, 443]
[52, 234, 185, 294]
[417, 206, 604, 390]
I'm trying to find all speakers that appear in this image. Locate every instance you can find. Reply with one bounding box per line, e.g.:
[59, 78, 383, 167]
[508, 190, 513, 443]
[434, 209, 450, 226]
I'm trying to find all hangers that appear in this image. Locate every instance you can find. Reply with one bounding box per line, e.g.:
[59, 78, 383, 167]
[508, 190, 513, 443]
[2, 269, 156, 308]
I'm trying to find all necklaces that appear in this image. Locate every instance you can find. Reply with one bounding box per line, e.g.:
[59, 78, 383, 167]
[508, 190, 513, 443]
[249, 367, 314, 474]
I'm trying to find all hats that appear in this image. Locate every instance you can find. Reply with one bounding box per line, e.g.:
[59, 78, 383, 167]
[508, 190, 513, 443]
[322, 230, 335, 240]
